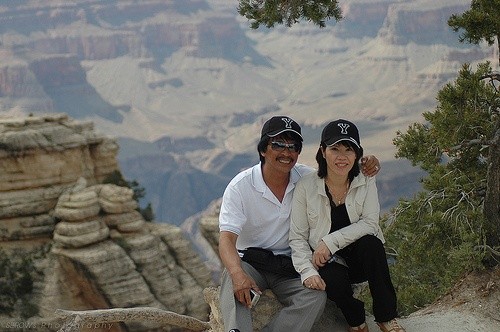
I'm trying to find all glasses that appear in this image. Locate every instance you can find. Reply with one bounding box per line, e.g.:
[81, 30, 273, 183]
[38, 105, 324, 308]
[266, 141, 299, 152]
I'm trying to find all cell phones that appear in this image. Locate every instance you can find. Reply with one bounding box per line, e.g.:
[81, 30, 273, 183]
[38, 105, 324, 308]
[244, 288, 260, 309]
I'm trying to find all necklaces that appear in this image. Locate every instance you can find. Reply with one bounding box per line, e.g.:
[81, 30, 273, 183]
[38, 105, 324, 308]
[325, 176, 350, 205]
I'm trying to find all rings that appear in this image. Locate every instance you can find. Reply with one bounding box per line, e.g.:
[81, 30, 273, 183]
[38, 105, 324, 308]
[374, 164, 380, 172]
[309, 283, 313, 288]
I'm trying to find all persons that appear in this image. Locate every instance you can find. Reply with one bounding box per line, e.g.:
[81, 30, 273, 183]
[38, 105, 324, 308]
[289, 119, 404, 332]
[217, 116, 383, 332]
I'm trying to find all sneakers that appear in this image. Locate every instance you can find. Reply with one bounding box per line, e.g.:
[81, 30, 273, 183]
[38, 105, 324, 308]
[375, 318, 407, 332]
[350, 322, 369, 332]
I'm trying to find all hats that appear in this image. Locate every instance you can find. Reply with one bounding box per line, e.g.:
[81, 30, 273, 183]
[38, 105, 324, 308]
[321, 119, 360, 149]
[261, 116, 304, 142]
